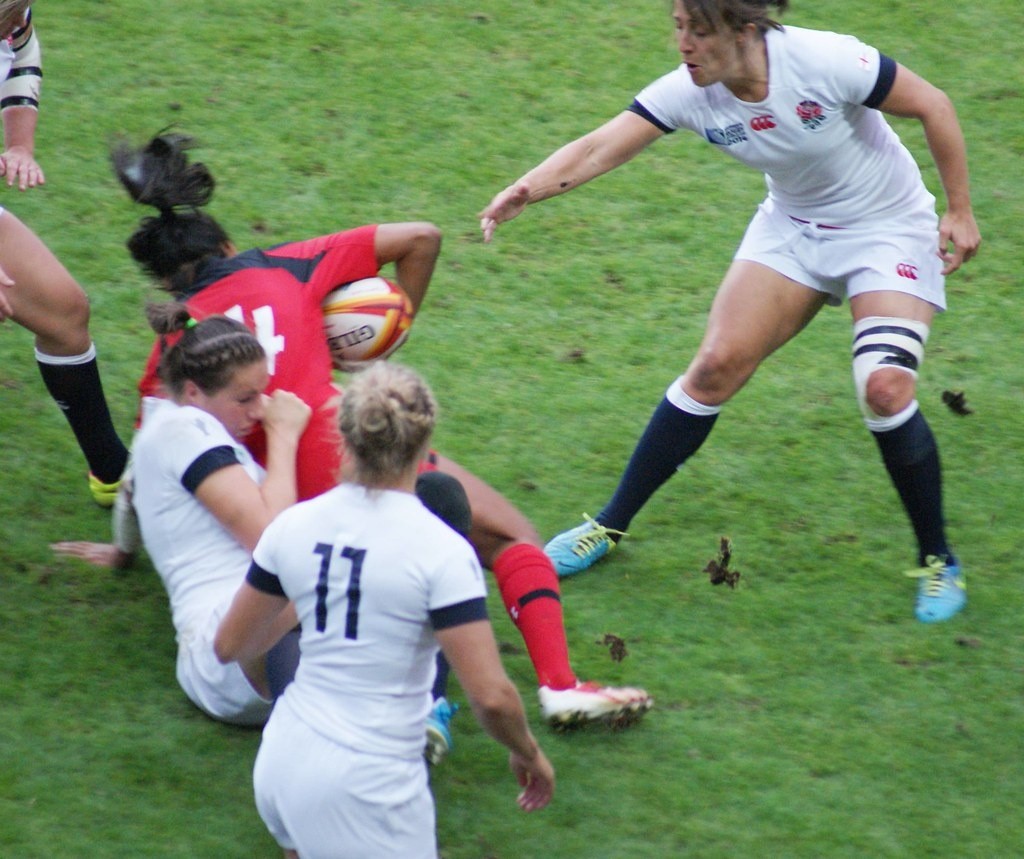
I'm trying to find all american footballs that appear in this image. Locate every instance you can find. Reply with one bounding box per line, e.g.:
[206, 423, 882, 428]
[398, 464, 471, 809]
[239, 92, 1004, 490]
[321, 274, 415, 371]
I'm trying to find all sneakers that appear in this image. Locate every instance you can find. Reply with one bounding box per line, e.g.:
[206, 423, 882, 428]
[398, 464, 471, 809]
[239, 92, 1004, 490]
[543, 512, 631, 576]
[537, 677, 653, 733]
[423, 697, 458, 766]
[902, 555, 968, 624]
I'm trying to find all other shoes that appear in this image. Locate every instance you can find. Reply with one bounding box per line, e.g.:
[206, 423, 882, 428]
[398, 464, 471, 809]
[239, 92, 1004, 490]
[89, 471, 124, 508]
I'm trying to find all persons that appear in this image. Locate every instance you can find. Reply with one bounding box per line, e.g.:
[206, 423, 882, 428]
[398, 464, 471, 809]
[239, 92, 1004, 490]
[0, 1, 129, 508]
[49, 118, 658, 858]
[475, 1, 985, 623]
